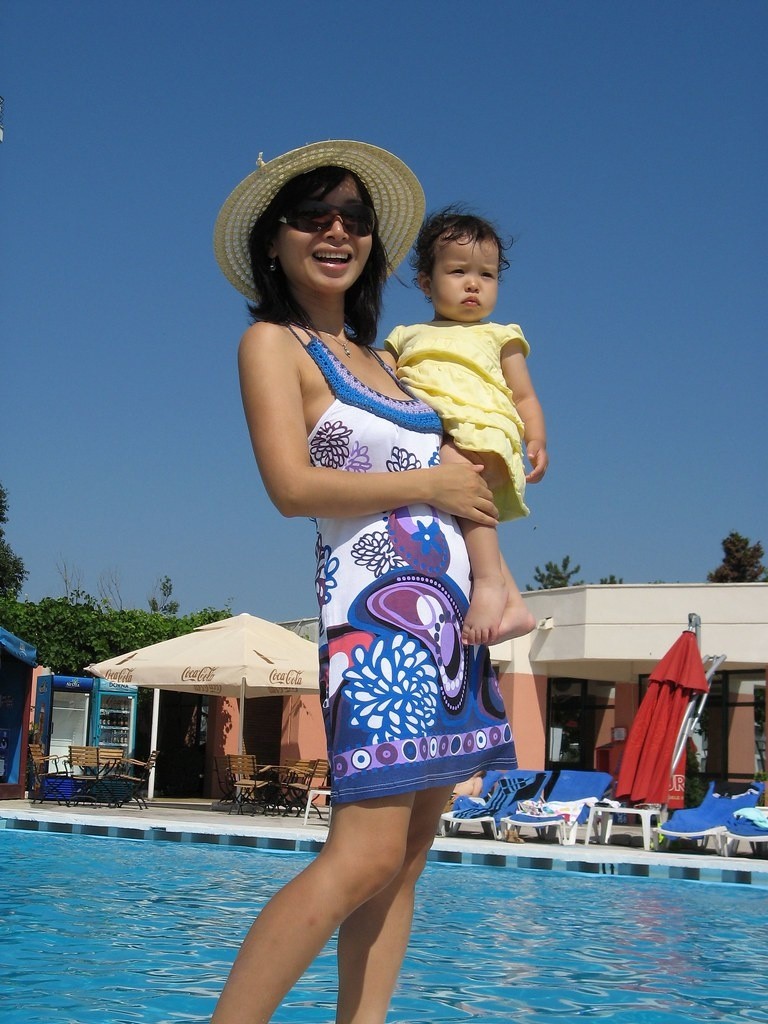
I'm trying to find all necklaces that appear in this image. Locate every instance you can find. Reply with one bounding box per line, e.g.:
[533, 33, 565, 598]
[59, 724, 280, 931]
[318, 331, 351, 357]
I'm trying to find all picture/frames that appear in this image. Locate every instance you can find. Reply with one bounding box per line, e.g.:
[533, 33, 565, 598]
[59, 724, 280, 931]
[611, 726, 628, 744]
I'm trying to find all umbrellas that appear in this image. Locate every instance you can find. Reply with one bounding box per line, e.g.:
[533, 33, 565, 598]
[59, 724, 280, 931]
[616, 628, 707, 822]
[84, 613, 320, 802]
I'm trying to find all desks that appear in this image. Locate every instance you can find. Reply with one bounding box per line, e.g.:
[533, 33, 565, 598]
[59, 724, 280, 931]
[586, 806, 661, 852]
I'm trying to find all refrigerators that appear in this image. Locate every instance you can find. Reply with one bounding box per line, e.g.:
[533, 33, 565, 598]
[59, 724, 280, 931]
[86, 677, 138, 803]
[29, 674, 95, 802]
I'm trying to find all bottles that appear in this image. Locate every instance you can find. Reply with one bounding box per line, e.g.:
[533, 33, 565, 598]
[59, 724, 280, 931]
[99, 729, 129, 744]
[0, 731, 9, 783]
[33, 704, 45, 744]
[100, 711, 130, 727]
[101, 695, 130, 710]
[0, 694, 16, 709]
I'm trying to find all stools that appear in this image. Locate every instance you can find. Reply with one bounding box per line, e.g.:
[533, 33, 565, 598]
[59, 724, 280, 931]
[305, 790, 332, 828]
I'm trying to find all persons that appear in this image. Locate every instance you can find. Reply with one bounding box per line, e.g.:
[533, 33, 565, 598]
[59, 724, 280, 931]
[213, 139, 518, 1024]
[384, 203, 549, 647]
[443, 771, 487, 813]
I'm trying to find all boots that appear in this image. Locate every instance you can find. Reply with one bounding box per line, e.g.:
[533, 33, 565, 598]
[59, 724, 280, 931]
[505, 829, 525, 843]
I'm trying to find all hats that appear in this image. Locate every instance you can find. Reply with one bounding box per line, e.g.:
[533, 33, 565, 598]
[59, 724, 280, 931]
[213, 138, 427, 303]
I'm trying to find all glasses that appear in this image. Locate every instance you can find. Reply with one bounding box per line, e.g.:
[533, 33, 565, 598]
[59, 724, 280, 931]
[273, 198, 378, 237]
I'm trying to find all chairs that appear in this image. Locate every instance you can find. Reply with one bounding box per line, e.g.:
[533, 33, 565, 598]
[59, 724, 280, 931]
[27, 744, 160, 810]
[214, 754, 330, 819]
[660, 781, 768, 857]
[440, 770, 610, 845]
